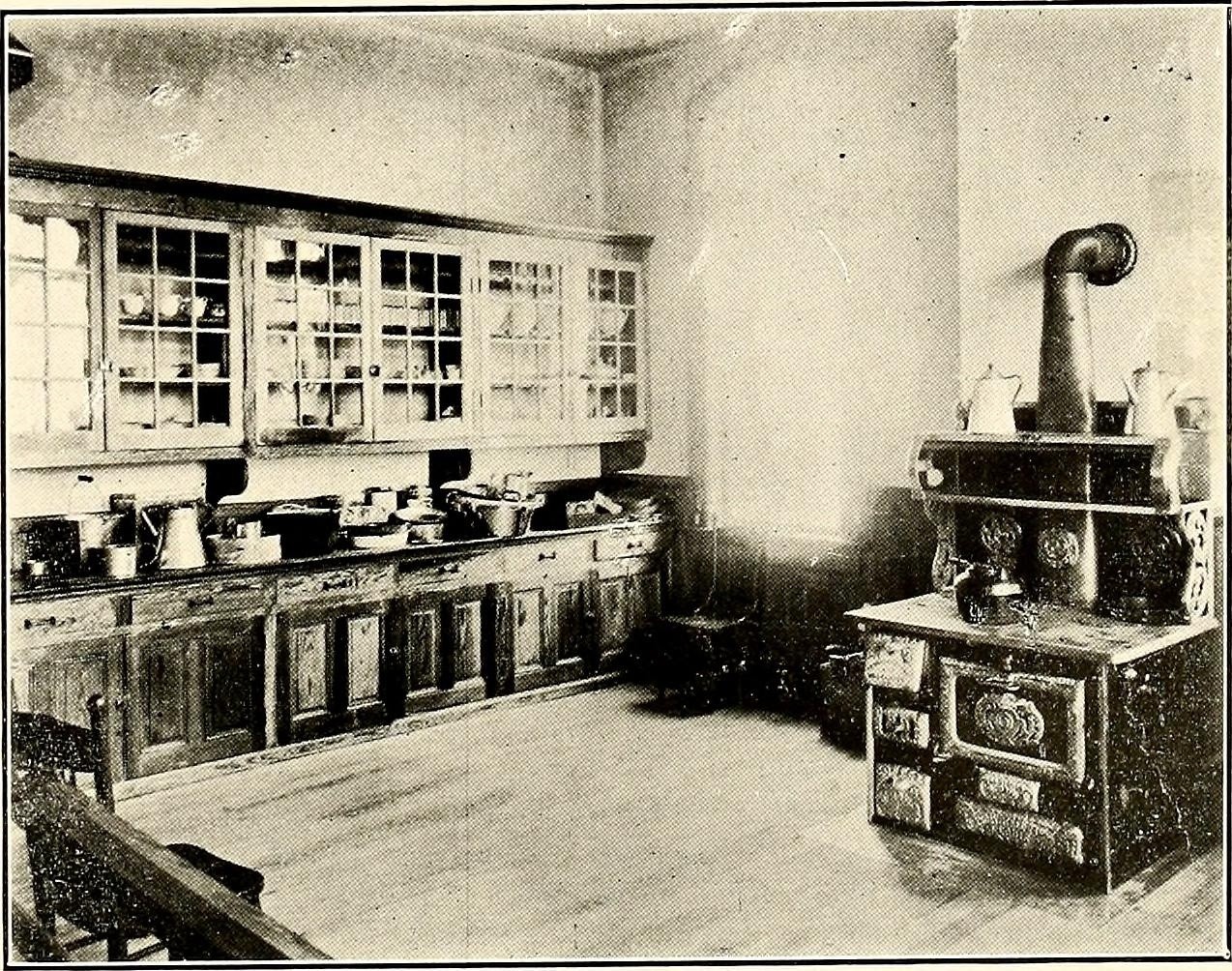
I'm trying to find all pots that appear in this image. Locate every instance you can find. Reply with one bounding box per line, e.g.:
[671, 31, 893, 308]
[443, 480, 547, 539]
[257, 508, 342, 558]
[338, 524, 408, 548]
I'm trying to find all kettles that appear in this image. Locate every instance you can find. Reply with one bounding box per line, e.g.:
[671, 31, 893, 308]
[943, 549, 1027, 627]
[141, 501, 209, 570]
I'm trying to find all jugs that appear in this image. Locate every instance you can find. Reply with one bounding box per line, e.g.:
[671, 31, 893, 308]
[956, 363, 1023, 436]
[1119, 359, 1180, 436]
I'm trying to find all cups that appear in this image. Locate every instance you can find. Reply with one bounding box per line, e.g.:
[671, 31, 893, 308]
[118, 294, 209, 318]
[22, 560, 46, 576]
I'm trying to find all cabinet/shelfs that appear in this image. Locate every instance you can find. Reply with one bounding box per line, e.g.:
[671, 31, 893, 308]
[9, 157, 655, 471]
[398, 543, 504, 734]
[277, 555, 398, 761]
[843, 587, 1222, 898]
[589, 519, 676, 690]
[125, 568, 277, 799]
[10, 585, 125, 805]
[503, 533, 592, 706]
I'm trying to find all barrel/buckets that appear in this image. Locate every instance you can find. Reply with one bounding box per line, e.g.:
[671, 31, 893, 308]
[87, 544, 137, 580]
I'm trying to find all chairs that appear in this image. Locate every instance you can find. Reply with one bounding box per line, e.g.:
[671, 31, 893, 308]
[656, 522, 767, 706]
[12, 711, 267, 963]
[817, 486, 936, 753]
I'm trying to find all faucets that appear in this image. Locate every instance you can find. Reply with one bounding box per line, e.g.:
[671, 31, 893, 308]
[503, 471, 533, 487]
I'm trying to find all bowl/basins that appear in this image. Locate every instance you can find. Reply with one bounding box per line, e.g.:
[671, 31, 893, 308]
[392, 508, 448, 543]
[119, 362, 221, 379]
